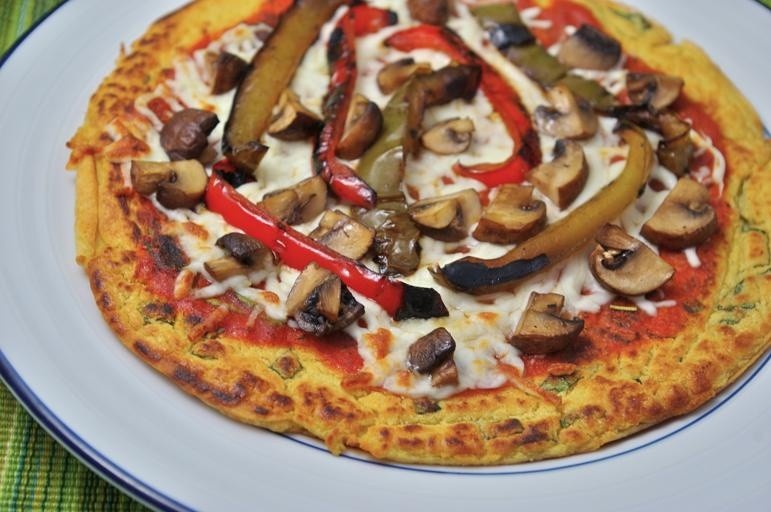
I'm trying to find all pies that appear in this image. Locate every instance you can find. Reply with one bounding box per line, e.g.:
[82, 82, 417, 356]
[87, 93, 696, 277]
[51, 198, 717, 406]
[68, 0, 771, 467]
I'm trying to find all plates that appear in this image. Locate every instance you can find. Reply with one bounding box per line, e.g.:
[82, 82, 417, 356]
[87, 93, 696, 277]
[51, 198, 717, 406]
[2, 0, 770, 510]
